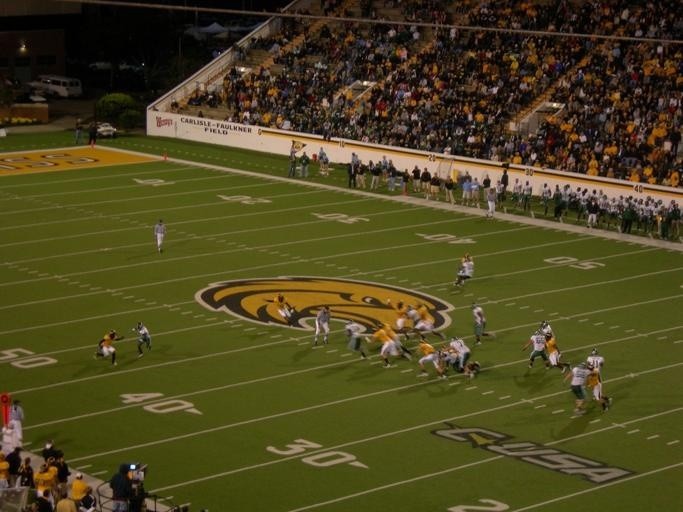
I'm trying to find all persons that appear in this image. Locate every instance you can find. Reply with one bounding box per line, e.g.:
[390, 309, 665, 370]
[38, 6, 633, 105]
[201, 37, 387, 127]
[0, 400, 210, 512]
[266, 293, 296, 324]
[132, 321, 152, 358]
[171, 0, 683, 246]
[74, 117, 83, 144]
[454, 255, 474, 288]
[87, 121, 97, 145]
[154, 220, 167, 252]
[345, 300, 612, 417]
[94, 329, 125, 366]
[314, 306, 331, 345]
